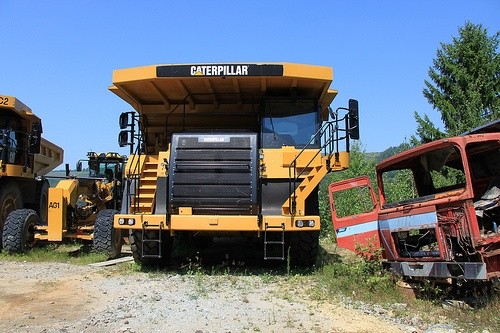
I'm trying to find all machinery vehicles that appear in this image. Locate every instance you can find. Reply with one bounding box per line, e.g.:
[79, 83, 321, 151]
[55, 150, 128, 240]
[93, 63, 359, 267]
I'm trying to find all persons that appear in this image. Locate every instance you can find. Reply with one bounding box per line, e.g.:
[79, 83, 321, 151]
[105, 169, 118, 183]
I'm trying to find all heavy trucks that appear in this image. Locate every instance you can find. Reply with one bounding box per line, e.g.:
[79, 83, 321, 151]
[0, 96, 62, 239]
